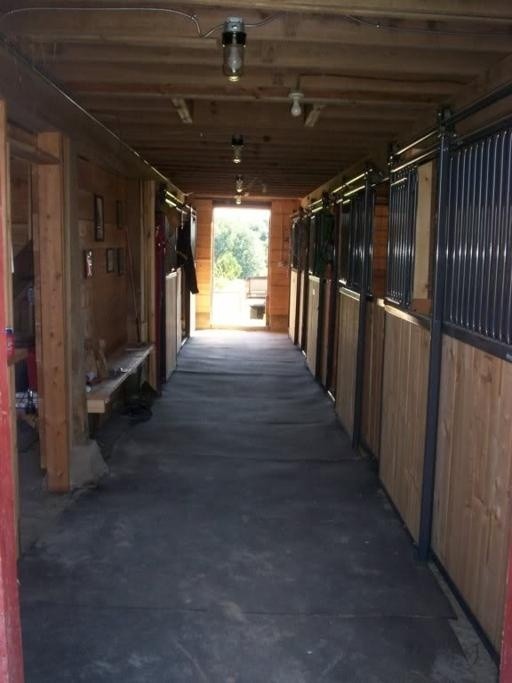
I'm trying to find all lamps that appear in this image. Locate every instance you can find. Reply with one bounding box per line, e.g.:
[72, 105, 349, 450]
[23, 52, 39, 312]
[288, 92, 305, 117]
[236, 194, 242, 205]
[219, 11, 253, 81]
[235, 173, 244, 192]
[231, 134, 244, 164]
[262, 183, 268, 193]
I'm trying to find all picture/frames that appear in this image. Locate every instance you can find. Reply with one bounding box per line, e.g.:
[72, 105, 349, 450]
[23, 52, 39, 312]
[107, 248, 114, 272]
[118, 248, 124, 275]
[94, 195, 104, 240]
[84, 249, 94, 278]
[116, 201, 123, 229]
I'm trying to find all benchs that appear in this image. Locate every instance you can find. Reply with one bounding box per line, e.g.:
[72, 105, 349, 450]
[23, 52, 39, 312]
[89, 343, 154, 439]
